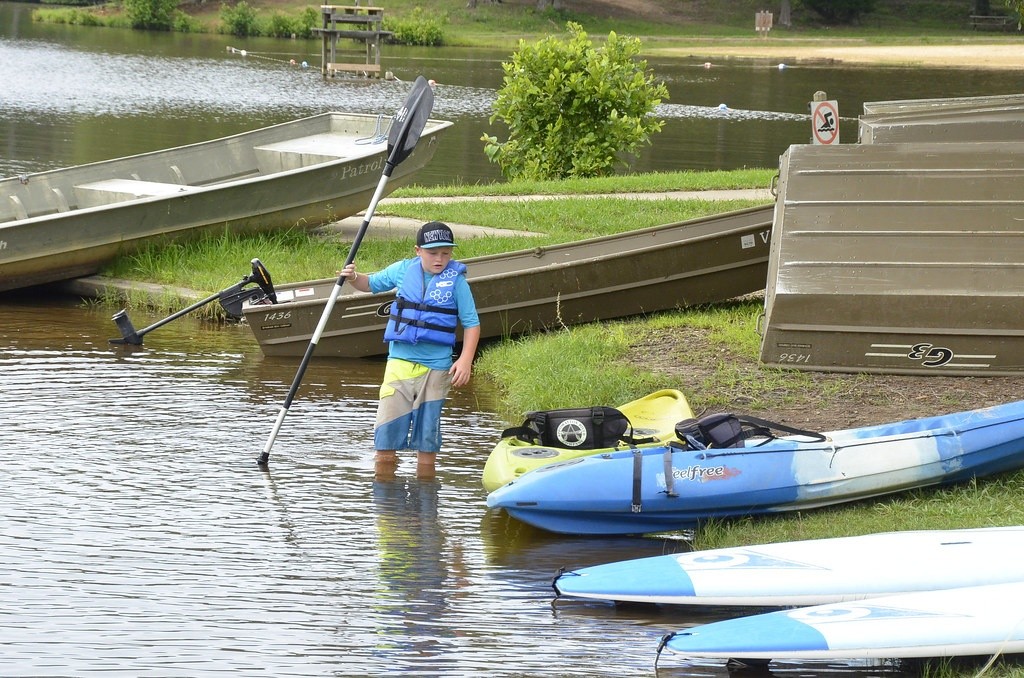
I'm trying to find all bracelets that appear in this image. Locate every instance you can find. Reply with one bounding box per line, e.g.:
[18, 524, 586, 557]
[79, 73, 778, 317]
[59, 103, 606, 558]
[348, 271, 357, 283]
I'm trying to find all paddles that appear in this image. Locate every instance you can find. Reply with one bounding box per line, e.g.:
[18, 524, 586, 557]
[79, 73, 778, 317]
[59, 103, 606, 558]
[256, 75, 435, 470]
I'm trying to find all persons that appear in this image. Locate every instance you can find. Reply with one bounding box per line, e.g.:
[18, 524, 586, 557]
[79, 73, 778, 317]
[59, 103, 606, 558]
[335, 222, 481, 465]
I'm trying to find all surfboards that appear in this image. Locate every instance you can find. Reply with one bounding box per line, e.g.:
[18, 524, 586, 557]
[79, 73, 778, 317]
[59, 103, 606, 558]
[657, 577, 1024, 662]
[553, 522, 1024, 607]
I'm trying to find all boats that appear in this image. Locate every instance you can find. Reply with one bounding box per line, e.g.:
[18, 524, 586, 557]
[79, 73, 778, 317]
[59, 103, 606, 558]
[756, 141, 1024, 376]
[479, 397, 1023, 534]
[856, 93, 1024, 146]
[109, 198, 779, 360]
[653, 582, 1024, 664]
[1, 112, 456, 304]
[541, 523, 1024, 604]
[480, 385, 698, 497]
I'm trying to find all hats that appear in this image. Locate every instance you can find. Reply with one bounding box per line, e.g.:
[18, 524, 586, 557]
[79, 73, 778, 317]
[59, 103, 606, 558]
[416, 221, 458, 249]
